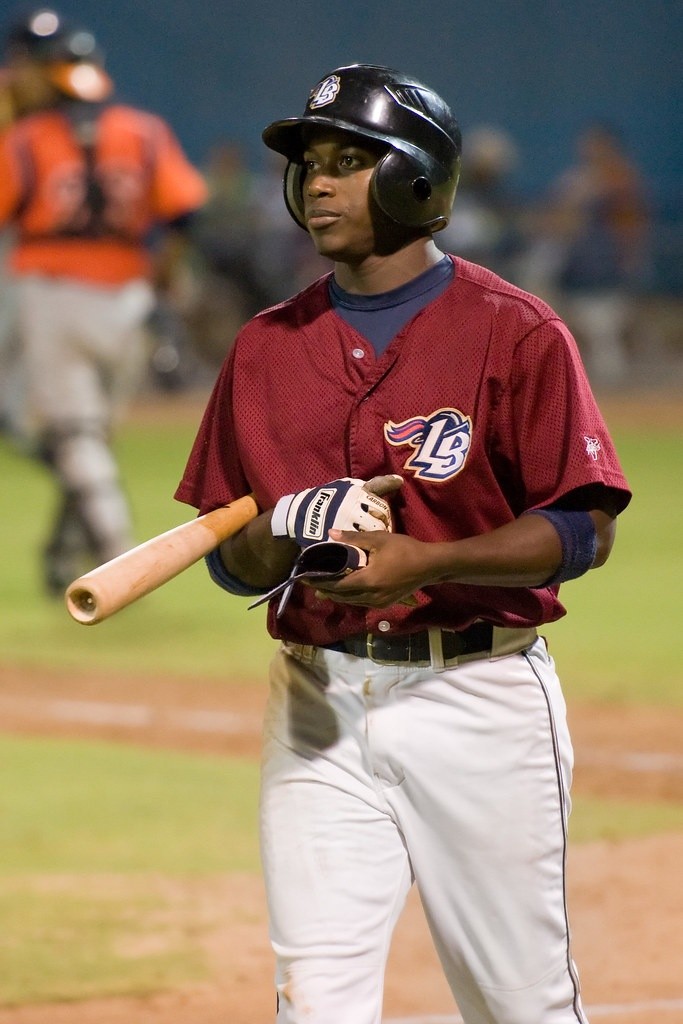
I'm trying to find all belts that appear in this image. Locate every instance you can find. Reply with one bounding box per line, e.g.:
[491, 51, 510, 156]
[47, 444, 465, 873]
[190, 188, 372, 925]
[318, 620, 494, 667]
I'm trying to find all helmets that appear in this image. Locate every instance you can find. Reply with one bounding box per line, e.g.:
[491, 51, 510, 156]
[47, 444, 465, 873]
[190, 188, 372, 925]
[263, 63, 462, 235]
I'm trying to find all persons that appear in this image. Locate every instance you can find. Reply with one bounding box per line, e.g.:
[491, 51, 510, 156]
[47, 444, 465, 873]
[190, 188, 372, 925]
[0, 18, 657, 599]
[172, 62, 632, 1024]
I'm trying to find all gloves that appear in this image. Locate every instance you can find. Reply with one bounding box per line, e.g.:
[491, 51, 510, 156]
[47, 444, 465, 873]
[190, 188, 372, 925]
[270, 474, 403, 552]
[247, 541, 418, 620]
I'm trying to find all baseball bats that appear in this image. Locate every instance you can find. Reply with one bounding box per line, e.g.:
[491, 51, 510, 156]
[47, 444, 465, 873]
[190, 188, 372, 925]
[64, 492, 263, 626]
